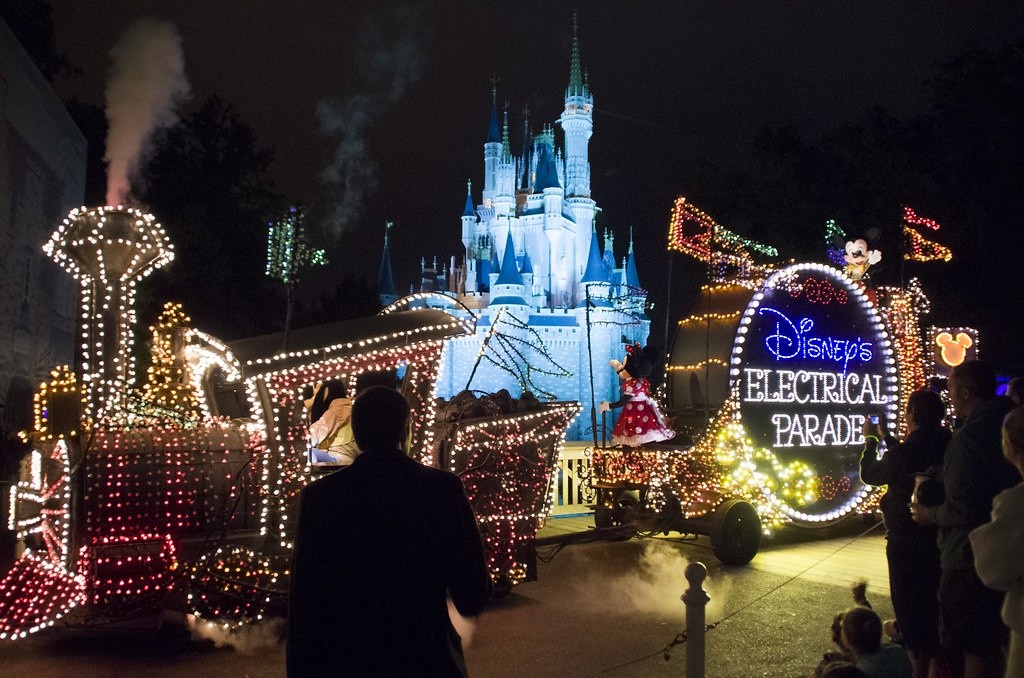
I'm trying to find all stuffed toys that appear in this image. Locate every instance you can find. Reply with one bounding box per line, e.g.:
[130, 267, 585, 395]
[596, 341, 674, 450]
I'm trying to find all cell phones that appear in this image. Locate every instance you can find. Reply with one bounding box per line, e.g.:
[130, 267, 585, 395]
[869, 415, 880, 424]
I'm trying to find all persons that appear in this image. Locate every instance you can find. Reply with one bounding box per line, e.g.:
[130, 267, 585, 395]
[808, 361, 1023, 678]
[286, 385, 491, 678]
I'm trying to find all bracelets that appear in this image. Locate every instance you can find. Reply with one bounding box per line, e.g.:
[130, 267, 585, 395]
[865, 434, 879, 443]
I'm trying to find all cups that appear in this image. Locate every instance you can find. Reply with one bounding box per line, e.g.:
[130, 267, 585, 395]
[911, 472, 935, 513]
